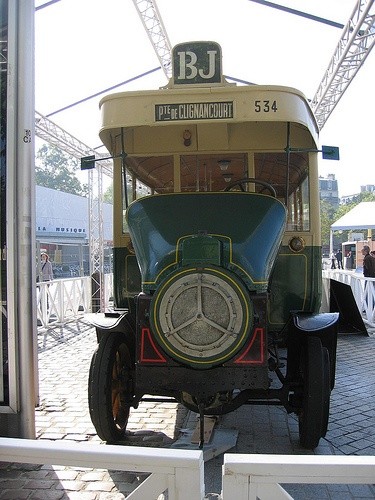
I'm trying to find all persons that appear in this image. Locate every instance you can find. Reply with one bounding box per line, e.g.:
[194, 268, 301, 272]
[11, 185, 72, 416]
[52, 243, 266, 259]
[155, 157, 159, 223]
[335, 250, 344, 269]
[370, 251, 375, 258]
[36, 253, 53, 313]
[361, 245, 375, 314]
[331, 251, 336, 269]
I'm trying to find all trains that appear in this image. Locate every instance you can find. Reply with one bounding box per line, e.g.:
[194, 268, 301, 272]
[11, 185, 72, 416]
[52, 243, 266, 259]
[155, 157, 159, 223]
[76, 40, 343, 449]
